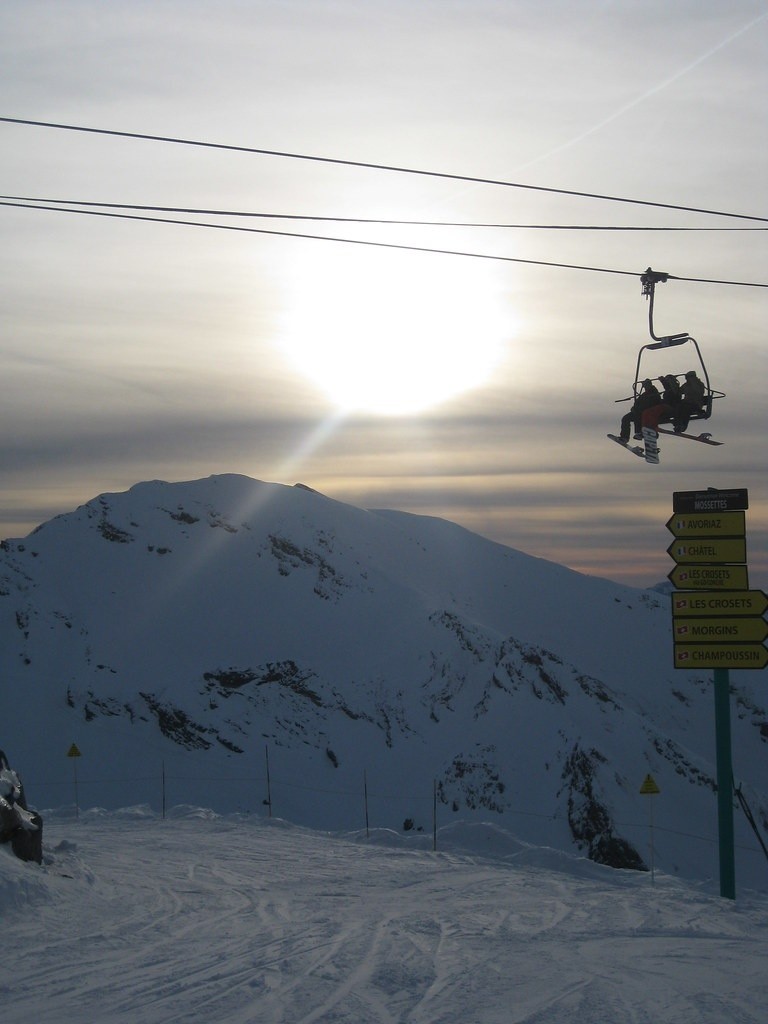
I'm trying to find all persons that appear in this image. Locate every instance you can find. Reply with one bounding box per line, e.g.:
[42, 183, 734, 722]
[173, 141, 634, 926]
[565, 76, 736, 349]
[673, 372, 705, 432]
[619, 373, 680, 444]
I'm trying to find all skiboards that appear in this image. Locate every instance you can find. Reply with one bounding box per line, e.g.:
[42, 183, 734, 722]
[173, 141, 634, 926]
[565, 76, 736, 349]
[607, 427, 723, 463]
